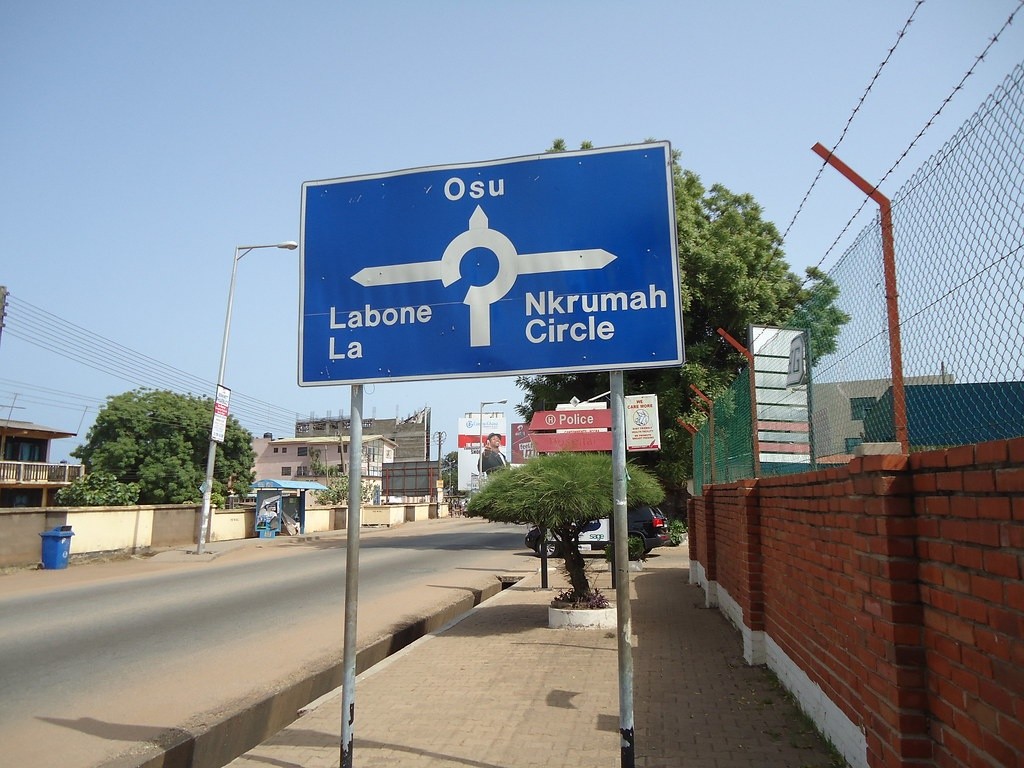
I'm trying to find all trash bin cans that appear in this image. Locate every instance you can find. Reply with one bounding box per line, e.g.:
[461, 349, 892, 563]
[37, 525, 75, 570]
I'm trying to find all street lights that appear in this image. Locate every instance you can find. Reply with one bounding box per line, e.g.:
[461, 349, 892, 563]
[196, 242, 300, 554]
[480, 399, 509, 490]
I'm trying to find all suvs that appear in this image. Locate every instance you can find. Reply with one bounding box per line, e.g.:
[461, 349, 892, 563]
[525, 495, 674, 562]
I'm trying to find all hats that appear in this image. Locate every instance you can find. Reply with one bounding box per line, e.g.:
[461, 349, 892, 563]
[488, 433, 501, 441]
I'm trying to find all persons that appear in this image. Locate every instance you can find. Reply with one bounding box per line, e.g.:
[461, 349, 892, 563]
[448, 498, 470, 518]
[477, 433, 511, 477]
